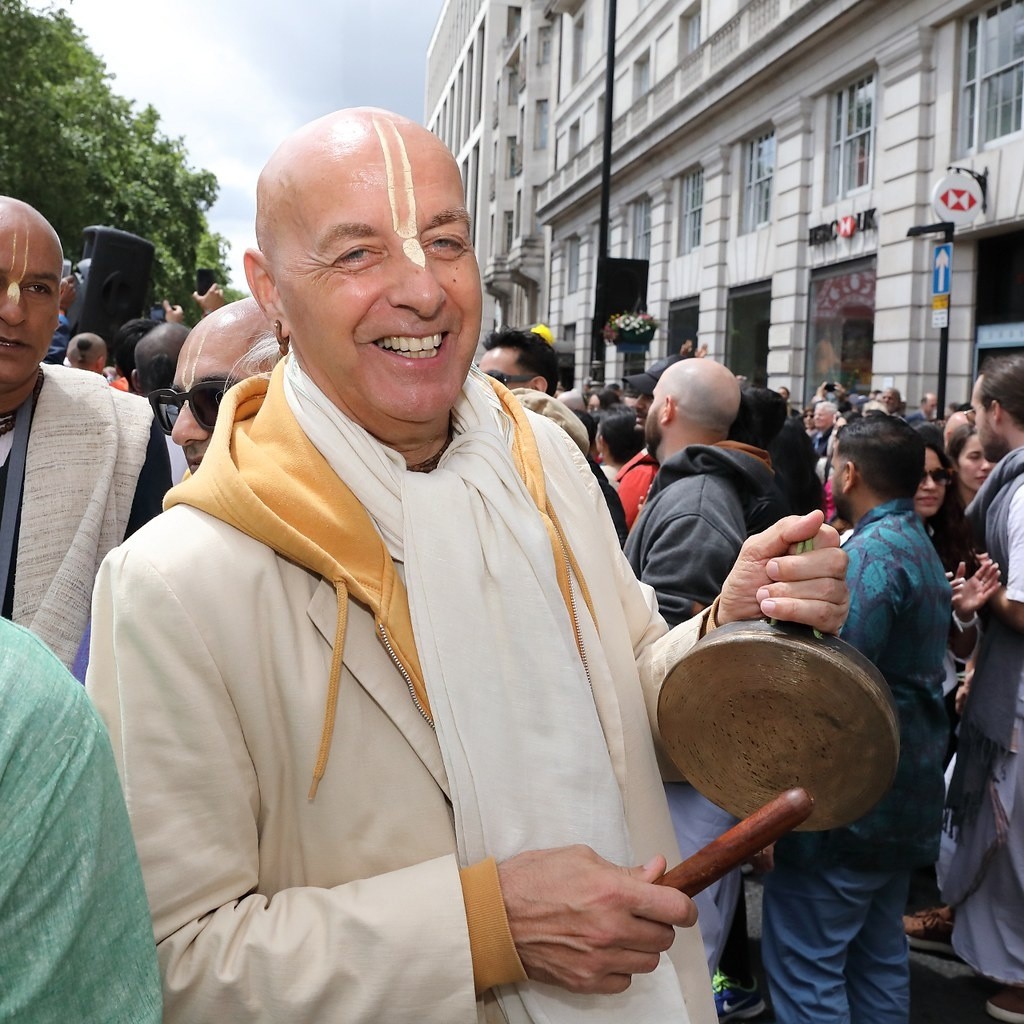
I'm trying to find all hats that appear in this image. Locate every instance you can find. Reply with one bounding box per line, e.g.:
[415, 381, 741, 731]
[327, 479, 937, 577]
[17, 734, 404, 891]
[621, 354, 687, 396]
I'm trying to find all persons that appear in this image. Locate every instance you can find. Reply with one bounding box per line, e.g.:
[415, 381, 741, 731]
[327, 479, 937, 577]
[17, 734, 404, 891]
[86, 107, 848, 1023]
[471, 325, 1024, 1024]
[0, 194, 277, 1024]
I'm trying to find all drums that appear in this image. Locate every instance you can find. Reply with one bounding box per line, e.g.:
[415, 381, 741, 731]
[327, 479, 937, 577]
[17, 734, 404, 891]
[655, 623, 903, 837]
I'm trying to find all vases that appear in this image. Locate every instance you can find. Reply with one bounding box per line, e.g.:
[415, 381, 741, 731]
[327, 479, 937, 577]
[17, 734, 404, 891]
[613, 328, 655, 347]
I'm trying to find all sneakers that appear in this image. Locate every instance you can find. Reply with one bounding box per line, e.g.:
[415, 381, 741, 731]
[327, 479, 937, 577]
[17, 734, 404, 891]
[712, 966, 766, 1024]
[901, 906, 956, 955]
[985, 985, 1024, 1024]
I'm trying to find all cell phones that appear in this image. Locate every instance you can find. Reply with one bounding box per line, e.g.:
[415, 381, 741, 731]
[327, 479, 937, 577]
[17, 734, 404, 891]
[197, 268, 215, 297]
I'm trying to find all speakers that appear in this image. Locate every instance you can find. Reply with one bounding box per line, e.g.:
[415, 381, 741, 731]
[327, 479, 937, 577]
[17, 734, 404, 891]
[68, 224, 155, 371]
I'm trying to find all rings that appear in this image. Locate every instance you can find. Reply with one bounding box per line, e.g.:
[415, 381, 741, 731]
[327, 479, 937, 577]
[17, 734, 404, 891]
[981, 579, 986, 583]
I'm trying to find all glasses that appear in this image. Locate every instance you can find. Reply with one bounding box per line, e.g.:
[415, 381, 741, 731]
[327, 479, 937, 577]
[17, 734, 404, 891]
[147, 378, 237, 437]
[919, 468, 950, 487]
[963, 403, 988, 422]
[485, 369, 534, 388]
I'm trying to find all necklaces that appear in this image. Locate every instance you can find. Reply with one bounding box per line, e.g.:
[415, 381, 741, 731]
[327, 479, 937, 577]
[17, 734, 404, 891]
[0, 367, 44, 435]
[406, 412, 454, 473]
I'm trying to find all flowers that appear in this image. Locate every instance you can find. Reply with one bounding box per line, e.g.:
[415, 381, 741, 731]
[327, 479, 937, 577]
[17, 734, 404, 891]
[606, 313, 658, 344]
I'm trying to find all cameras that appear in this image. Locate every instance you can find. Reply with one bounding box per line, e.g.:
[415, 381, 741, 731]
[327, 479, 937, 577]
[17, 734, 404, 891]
[151, 303, 175, 322]
